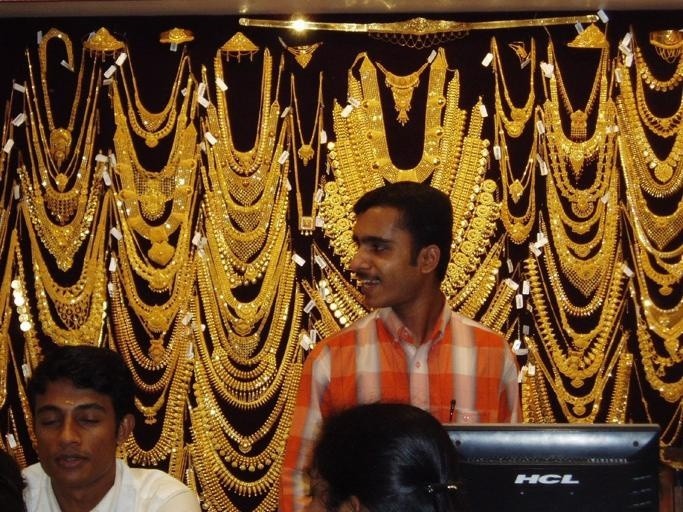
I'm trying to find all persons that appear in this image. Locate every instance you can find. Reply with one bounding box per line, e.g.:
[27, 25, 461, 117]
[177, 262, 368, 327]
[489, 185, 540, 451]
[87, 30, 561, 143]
[299, 400, 466, 511]
[18, 344, 199, 511]
[0, 447, 28, 511]
[273, 178, 521, 511]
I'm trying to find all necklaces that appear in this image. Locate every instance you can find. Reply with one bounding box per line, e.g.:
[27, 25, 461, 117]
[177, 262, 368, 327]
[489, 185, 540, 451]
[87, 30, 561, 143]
[185, 476, 280, 511]
[0, 0, 683, 512]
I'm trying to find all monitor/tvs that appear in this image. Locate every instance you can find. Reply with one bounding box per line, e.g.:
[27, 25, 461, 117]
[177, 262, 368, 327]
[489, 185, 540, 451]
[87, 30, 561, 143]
[442, 424, 660, 512]
